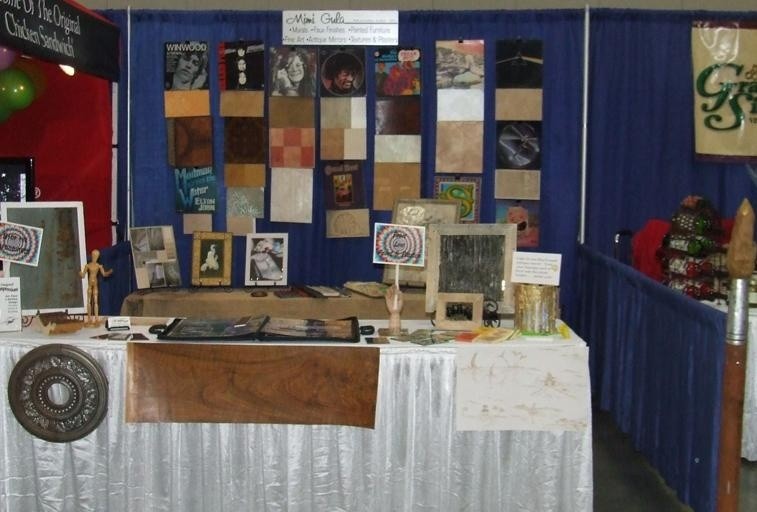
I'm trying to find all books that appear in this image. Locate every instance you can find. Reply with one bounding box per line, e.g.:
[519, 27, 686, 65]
[436, 327, 519, 343]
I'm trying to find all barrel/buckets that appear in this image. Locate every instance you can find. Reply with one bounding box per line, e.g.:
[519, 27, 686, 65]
[514, 285, 559, 337]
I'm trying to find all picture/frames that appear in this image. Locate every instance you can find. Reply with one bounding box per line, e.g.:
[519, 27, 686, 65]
[424, 223, 517, 320]
[1, 197, 92, 317]
[244, 232, 288, 289]
[431, 293, 482, 332]
[381, 197, 462, 289]
[191, 230, 231, 289]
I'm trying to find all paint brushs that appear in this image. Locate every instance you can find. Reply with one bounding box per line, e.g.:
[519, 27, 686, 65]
[718, 196, 756, 512]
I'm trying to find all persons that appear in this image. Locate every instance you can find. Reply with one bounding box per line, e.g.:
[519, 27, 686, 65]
[270, 47, 316, 96]
[323, 53, 358, 95]
[234, 45, 251, 91]
[376, 61, 419, 95]
[205, 244, 219, 272]
[385, 284, 404, 334]
[166, 41, 209, 90]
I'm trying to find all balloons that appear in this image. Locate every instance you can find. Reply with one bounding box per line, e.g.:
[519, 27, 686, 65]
[0, 44, 45, 123]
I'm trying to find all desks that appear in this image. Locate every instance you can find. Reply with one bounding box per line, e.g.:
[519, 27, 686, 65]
[120, 289, 432, 318]
[0, 317, 595, 511]
[703, 297, 756, 464]
